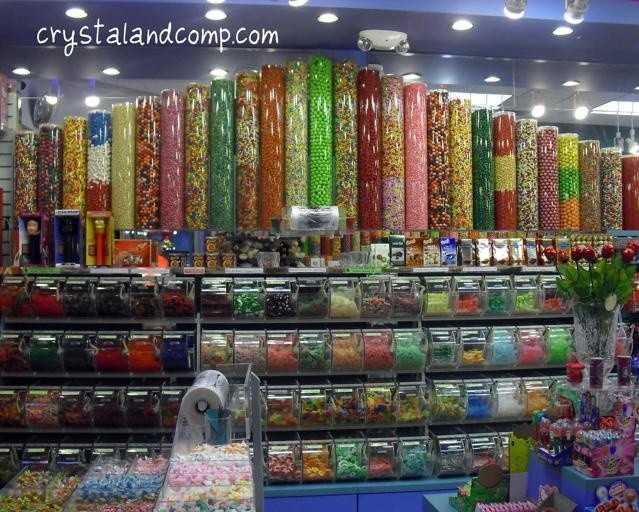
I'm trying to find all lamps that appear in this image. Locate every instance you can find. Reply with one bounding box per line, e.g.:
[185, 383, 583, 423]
[566, 2, 588, 25]
[502, 0, 527, 21]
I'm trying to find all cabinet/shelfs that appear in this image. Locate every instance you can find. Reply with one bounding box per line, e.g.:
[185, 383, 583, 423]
[1, 228, 639, 512]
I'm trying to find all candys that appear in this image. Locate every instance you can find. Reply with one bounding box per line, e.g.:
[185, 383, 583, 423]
[0, 56, 639, 512]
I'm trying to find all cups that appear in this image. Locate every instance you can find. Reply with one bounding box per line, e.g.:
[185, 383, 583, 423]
[590, 337, 633, 388]
[348, 251, 366, 267]
[257, 252, 281, 268]
